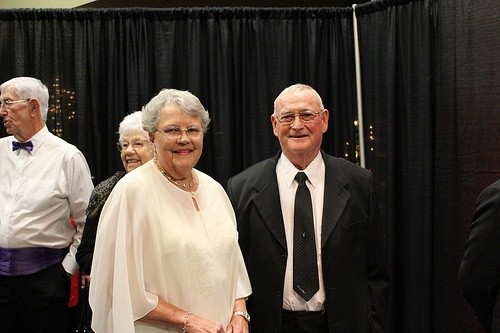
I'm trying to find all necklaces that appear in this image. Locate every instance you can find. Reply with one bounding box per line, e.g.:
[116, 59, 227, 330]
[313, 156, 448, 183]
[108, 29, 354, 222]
[153, 157, 195, 188]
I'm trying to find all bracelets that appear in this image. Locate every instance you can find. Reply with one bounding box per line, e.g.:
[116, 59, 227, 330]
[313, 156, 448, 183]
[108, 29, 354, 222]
[182, 312, 191, 333]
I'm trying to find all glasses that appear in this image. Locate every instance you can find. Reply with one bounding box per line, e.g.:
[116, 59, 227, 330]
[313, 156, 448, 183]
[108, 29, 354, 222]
[275, 110, 323, 124]
[155, 126, 204, 137]
[0, 99, 30, 107]
[117, 139, 150, 152]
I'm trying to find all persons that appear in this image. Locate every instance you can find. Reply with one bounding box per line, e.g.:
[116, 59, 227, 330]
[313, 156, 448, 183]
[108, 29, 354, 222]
[75, 109, 154, 275]
[228, 83, 388, 333]
[88, 88, 253, 333]
[0, 77, 94, 333]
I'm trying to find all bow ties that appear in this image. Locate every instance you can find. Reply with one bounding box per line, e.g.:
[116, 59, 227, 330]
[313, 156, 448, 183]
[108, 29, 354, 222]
[11, 141, 33, 153]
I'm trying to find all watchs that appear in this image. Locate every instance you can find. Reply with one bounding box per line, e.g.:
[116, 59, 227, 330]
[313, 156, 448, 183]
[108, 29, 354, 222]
[231, 311, 251, 324]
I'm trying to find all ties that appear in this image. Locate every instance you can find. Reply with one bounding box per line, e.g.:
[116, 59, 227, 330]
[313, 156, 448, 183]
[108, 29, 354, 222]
[292, 172, 320, 302]
